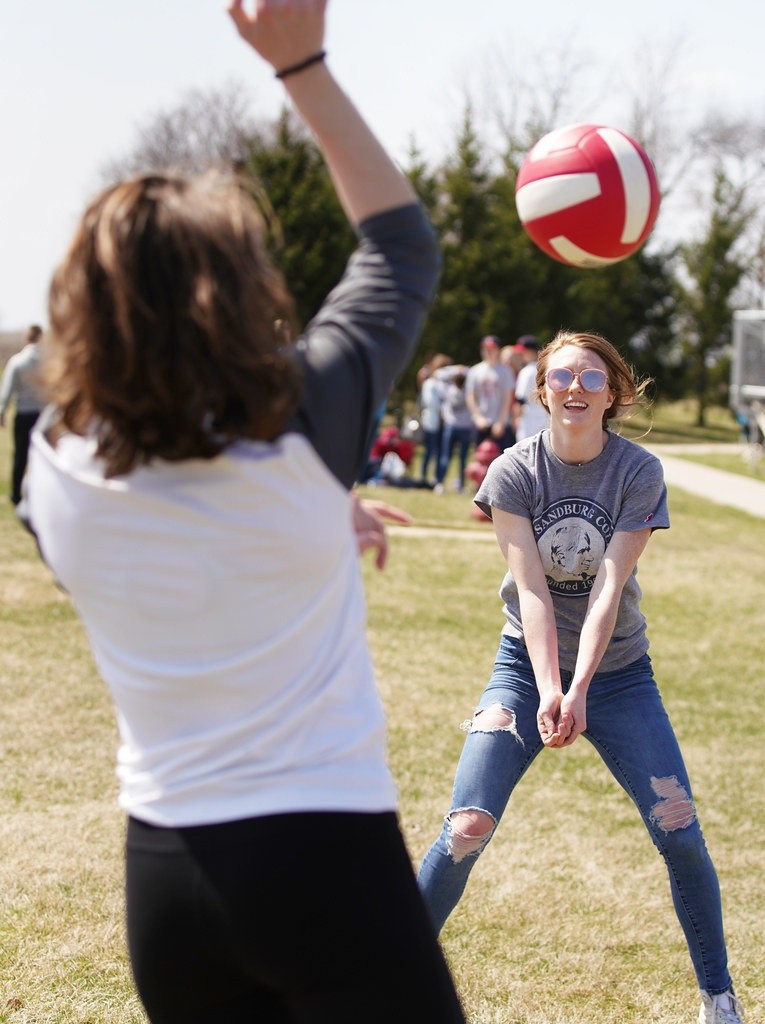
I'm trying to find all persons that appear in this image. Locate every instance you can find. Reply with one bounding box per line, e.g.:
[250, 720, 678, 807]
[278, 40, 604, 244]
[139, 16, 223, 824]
[413, 331, 738, 1023]
[15, 0, 466, 1024]
[0, 324, 47, 505]
[356, 336, 550, 487]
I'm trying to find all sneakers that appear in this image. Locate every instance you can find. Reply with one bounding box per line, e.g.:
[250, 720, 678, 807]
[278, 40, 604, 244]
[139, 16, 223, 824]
[431, 477, 445, 494]
[689, 986, 746, 1024]
[421, 475, 426, 479]
[456, 485, 468, 494]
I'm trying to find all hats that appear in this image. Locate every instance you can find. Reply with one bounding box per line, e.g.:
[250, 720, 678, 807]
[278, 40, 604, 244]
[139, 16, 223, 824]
[479, 335, 501, 350]
[512, 335, 540, 352]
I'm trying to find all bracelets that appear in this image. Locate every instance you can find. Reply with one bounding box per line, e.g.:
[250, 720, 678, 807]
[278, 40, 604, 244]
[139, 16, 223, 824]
[275, 50, 326, 78]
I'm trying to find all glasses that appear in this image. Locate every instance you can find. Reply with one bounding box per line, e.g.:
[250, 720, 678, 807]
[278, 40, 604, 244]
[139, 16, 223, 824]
[543, 367, 609, 394]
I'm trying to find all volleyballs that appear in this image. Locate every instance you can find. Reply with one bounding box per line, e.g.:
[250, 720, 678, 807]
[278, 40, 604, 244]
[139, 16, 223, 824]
[516, 123, 659, 266]
